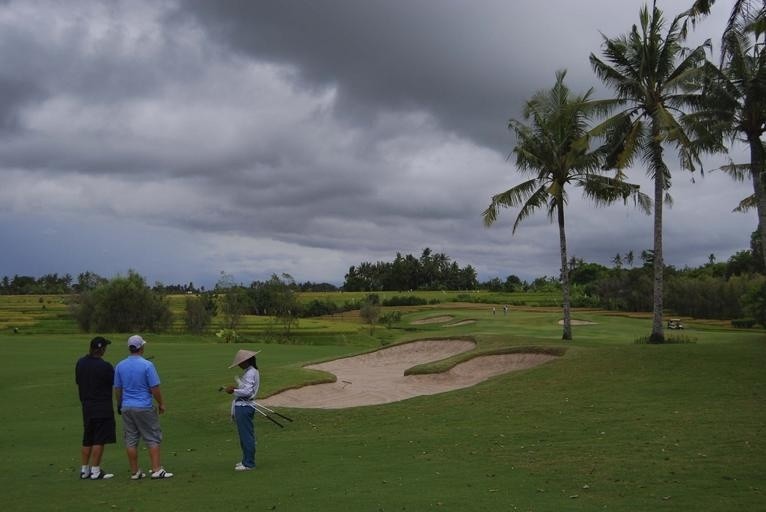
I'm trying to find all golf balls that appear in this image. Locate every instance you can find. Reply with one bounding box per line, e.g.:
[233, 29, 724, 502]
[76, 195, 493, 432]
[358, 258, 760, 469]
[149, 469, 152, 473]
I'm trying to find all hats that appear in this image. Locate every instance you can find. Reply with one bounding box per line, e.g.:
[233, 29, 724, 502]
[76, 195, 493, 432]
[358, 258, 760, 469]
[127, 334, 147, 352]
[90, 336, 112, 349]
[227, 348, 264, 369]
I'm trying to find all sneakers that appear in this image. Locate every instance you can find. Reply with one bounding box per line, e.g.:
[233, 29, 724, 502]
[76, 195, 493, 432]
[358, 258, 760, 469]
[149, 469, 174, 480]
[80, 471, 91, 480]
[234, 465, 256, 472]
[89, 469, 115, 480]
[236, 462, 243, 467]
[130, 467, 147, 480]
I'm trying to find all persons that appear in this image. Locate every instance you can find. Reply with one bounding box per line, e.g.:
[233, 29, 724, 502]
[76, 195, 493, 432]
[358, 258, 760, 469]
[112, 335, 173, 481]
[74, 335, 114, 482]
[222, 347, 259, 473]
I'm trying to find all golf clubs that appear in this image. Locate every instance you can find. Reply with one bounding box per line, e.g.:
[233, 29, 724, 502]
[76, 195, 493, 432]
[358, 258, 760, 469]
[219, 385, 293, 428]
